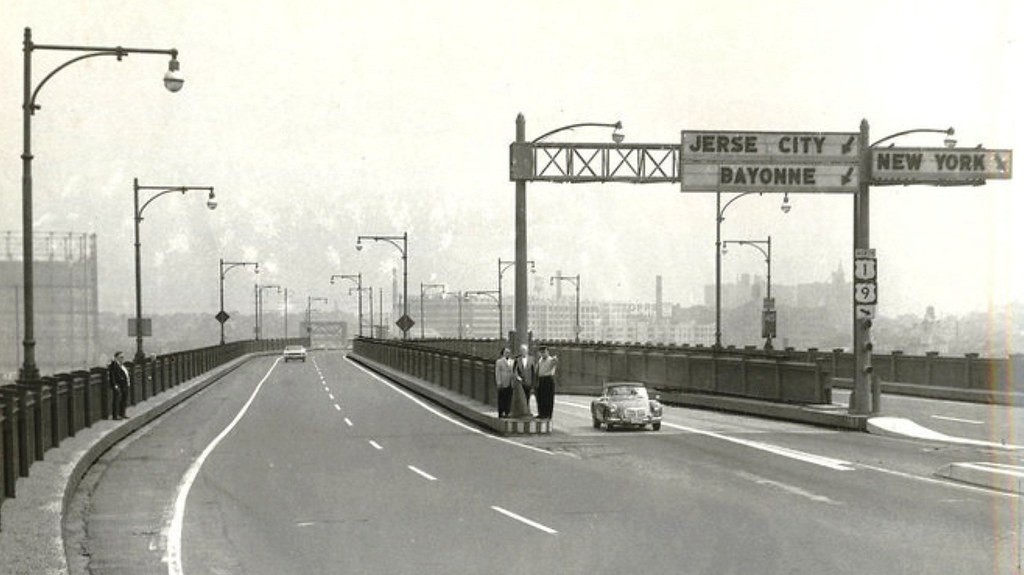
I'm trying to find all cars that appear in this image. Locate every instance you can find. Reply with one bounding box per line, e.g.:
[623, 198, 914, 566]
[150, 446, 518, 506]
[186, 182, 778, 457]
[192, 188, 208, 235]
[590, 380, 663, 432]
[283, 345, 309, 362]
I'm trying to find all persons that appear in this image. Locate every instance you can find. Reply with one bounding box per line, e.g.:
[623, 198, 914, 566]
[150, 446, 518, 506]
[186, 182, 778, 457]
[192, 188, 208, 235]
[108, 351, 130, 420]
[495, 347, 514, 419]
[534, 345, 559, 420]
[513, 345, 536, 401]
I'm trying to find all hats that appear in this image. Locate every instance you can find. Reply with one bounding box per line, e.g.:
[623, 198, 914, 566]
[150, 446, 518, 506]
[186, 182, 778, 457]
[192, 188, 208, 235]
[538, 346, 547, 350]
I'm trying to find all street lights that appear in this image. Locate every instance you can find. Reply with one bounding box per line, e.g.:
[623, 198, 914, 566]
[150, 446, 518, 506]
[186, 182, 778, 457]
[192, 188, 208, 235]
[221, 263, 261, 344]
[348, 288, 375, 340]
[22, 42, 186, 384]
[308, 297, 328, 338]
[715, 189, 792, 349]
[515, 122, 626, 370]
[356, 235, 409, 340]
[420, 285, 446, 338]
[330, 275, 362, 337]
[550, 276, 579, 343]
[443, 260, 538, 339]
[133, 185, 220, 362]
[256, 285, 283, 343]
[850, 123, 958, 413]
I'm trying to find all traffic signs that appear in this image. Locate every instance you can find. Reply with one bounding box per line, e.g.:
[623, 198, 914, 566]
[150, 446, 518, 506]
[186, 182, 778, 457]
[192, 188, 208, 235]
[680, 130, 860, 195]
